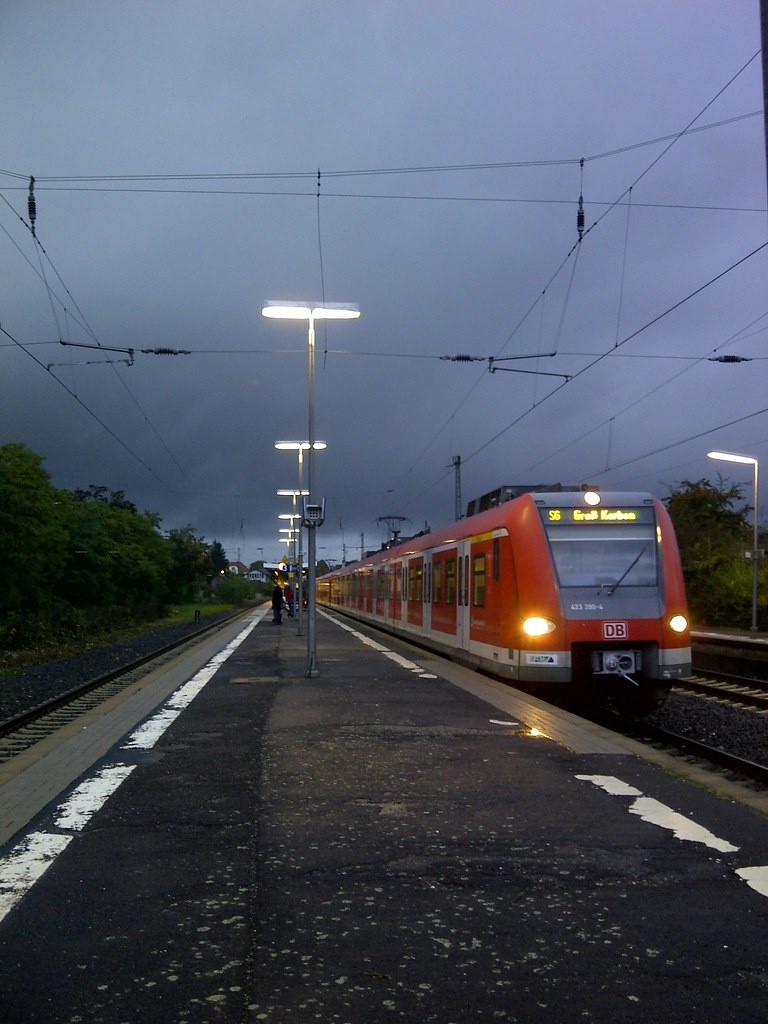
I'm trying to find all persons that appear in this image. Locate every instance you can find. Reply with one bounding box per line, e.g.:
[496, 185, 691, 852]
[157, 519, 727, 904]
[285, 585, 309, 617]
[272, 581, 284, 625]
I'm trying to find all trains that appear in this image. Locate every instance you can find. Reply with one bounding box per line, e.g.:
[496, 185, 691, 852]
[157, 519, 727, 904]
[314, 490, 693, 718]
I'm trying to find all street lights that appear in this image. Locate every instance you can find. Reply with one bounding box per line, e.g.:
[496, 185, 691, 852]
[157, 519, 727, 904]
[272, 438, 328, 638]
[275, 488, 310, 621]
[263, 300, 363, 681]
[707, 449, 760, 634]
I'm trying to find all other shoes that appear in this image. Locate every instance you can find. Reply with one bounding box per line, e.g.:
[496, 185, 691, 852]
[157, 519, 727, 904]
[288, 613, 293, 617]
[272, 622, 279, 625]
